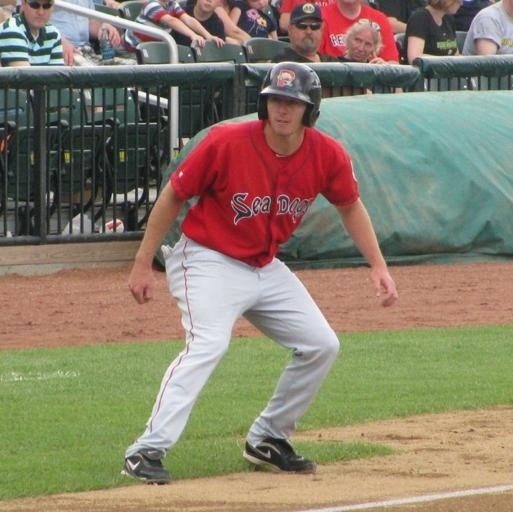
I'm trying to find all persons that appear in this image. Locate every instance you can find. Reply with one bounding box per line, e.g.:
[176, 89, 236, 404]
[122, 61, 397, 484]
[0, 1, 513, 115]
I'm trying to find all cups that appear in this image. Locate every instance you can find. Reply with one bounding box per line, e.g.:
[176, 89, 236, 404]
[98, 219, 123, 235]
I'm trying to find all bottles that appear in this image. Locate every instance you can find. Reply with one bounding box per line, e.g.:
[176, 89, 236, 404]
[97, 27, 113, 65]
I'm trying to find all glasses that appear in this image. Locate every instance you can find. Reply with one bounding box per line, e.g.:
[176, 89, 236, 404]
[26, 1, 54, 10]
[295, 22, 321, 30]
[353, 18, 382, 32]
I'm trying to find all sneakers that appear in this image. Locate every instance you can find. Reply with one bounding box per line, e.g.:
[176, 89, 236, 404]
[120, 448, 170, 484]
[243, 437, 315, 474]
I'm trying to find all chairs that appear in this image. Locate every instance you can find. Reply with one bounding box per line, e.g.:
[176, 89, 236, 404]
[0, 82, 177, 242]
[93, 0, 510, 142]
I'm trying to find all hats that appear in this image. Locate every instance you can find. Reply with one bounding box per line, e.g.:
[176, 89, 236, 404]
[290, 3, 323, 23]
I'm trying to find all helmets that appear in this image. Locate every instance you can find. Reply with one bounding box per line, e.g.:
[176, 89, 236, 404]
[256, 60, 322, 127]
